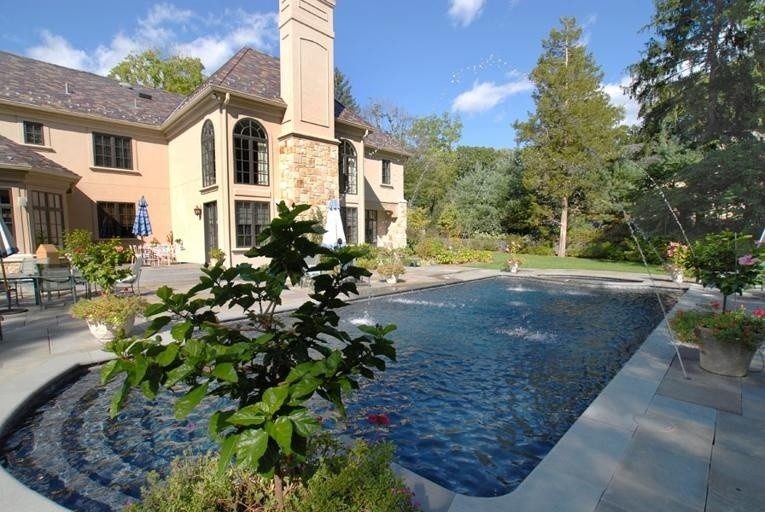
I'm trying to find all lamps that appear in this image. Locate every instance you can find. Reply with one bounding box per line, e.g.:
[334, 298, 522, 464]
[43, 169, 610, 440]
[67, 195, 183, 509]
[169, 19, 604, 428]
[194, 205, 202, 216]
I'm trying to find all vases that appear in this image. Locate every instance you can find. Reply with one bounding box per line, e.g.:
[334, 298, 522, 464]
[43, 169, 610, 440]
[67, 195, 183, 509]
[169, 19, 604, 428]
[508, 262, 519, 272]
[85, 315, 135, 344]
[695, 326, 761, 378]
[668, 268, 685, 281]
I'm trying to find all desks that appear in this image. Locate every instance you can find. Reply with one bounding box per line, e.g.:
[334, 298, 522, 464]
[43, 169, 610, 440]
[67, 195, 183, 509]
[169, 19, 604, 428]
[0, 274, 40, 305]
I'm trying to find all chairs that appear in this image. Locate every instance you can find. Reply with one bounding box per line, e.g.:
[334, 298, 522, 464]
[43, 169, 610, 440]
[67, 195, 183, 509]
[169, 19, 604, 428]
[16, 241, 182, 304]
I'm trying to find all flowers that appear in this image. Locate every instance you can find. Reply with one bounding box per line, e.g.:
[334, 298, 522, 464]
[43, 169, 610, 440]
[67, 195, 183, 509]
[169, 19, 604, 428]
[69, 288, 152, 327]
[502, 240, 526, 266]
[663, 240, 691, 268]
[667, 300, 765, 344]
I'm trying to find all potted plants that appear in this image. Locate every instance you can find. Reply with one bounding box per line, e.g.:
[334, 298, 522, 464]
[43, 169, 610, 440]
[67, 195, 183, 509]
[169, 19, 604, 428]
[376, 246, 407, 284]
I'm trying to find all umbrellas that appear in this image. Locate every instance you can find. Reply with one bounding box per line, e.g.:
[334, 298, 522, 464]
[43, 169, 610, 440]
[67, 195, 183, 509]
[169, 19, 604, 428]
[0, 215, 20, 296]
[321, 196, 348, 252]
[131, 194, 153, 257]
